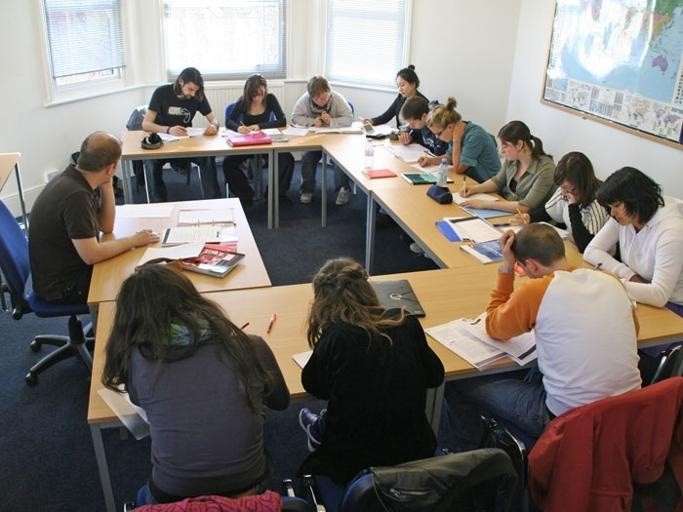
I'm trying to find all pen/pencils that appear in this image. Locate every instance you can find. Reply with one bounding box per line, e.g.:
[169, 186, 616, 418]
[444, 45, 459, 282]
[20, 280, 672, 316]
[267, 313, 276, 334]
[240, 322, 249, 332]
[240, 121, 245, 127]
[594, 262, 603, 269]
[463, 176, 466, 195]
[135, 230, 159, 234]
[408, 129, 413, 136]
[516, 208, 527, 225]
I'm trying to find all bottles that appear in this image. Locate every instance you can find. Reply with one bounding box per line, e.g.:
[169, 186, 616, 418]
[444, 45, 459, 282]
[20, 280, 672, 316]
[362, 138, 374, 171]
[437, 158, 448, 189]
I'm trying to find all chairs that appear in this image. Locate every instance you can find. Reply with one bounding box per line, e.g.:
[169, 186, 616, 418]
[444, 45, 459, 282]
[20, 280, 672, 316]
[0, 199, 96, 386]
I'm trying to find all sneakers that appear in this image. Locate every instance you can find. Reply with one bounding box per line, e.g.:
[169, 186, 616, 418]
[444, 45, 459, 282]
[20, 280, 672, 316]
[335, 190, 352, 206]
[299, 407, 324, 453]
[300, 191, 314, 204]
[239, 191, 254, 205]
[264, 191, 294, 205]
[410, 243, 424, 254]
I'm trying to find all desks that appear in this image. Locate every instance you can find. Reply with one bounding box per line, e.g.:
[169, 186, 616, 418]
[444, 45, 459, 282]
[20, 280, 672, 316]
[0, 152, 27, 239]
[88, 242, 682, 508]
[116, 124, 575, 242]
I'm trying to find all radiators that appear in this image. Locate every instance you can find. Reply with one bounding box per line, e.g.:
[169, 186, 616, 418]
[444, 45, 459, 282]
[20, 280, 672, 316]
[185, 82, 286, 127]
